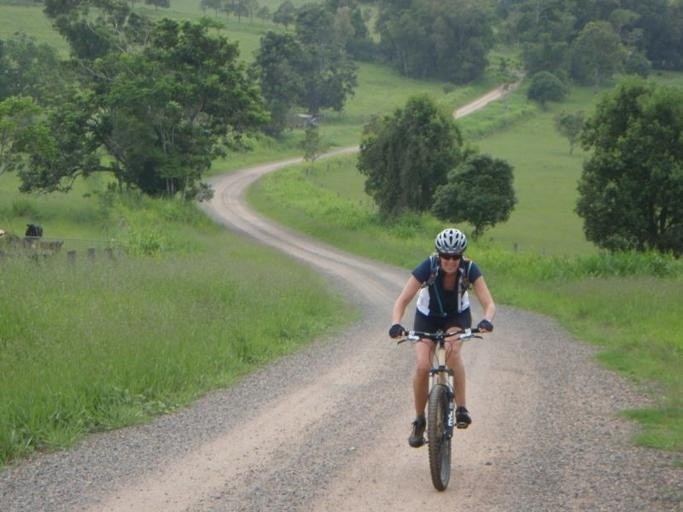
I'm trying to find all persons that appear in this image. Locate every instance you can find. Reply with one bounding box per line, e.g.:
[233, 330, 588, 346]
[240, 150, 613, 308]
[389, 228, 497, 447]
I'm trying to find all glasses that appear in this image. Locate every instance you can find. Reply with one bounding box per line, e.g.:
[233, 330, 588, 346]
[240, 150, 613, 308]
[439, 253, 463, 261]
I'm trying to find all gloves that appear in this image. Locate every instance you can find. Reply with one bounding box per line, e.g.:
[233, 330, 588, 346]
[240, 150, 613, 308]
[477, 318, 496, 335]
[387, 325, 411, 339]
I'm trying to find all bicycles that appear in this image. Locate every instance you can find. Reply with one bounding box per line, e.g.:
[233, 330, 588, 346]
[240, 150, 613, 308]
[395, 326, 487, 496]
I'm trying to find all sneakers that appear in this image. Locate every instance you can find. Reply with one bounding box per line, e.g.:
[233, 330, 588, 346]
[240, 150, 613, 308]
[408, 417, 427, 448]
[453, 406, 473, 428]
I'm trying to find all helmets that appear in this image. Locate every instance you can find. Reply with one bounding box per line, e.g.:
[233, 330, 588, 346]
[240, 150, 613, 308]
[434, 228, 466, 253]
